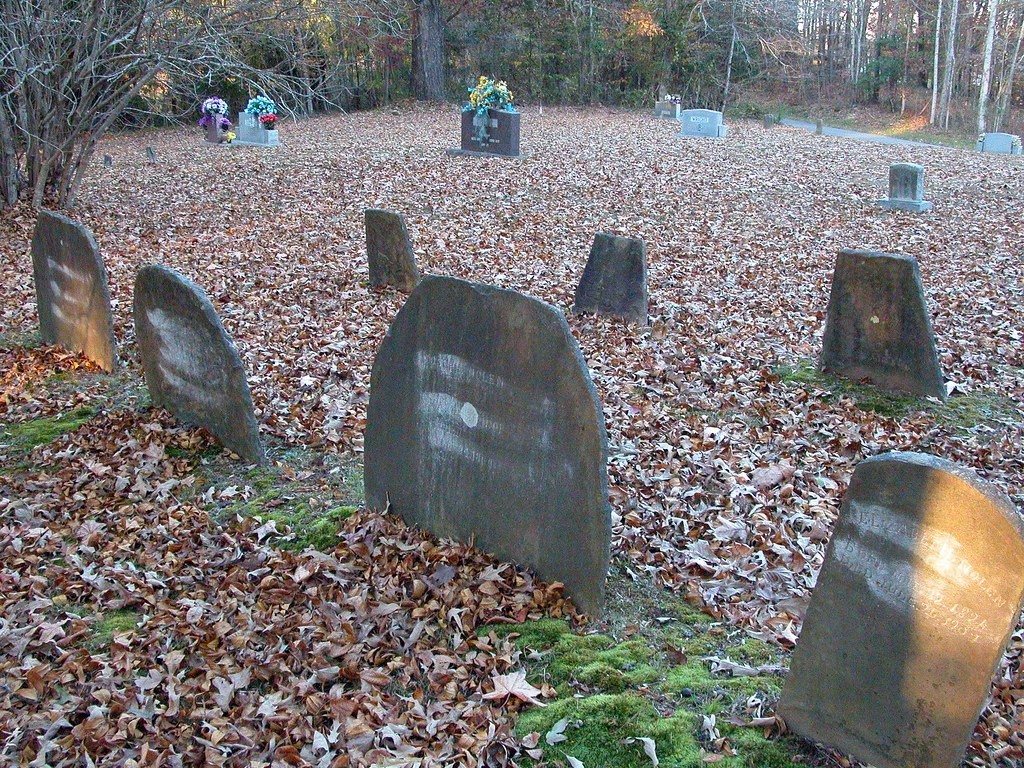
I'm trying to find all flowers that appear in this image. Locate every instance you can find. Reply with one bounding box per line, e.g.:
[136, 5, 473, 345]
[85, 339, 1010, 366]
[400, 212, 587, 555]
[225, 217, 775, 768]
[460, 75, 514, 151]
[664, 93, 682, 105]
[198, 96, 278, 144]
